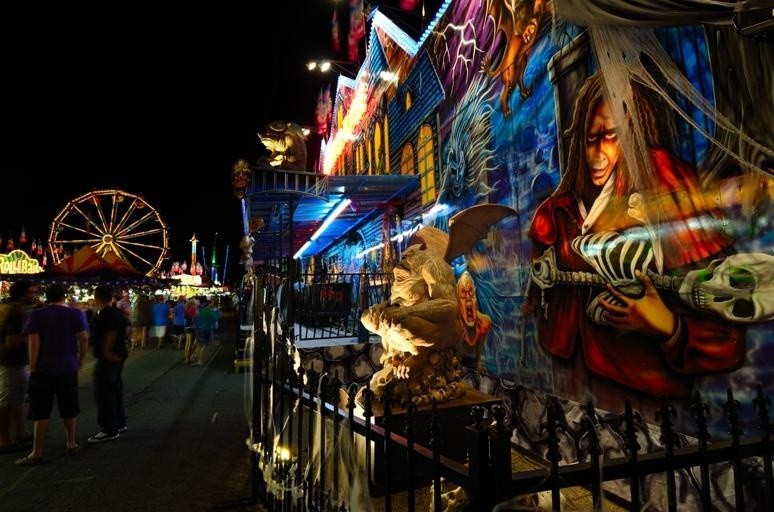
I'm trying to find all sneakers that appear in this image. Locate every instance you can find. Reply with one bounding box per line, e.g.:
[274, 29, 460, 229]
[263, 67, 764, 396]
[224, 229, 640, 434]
[87, 425, 127, 442]
[14, 456, 42, 466]
[66, 441, 83, 455]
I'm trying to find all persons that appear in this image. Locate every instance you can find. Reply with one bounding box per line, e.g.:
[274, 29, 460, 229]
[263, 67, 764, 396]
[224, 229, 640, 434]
[452, 271, 492, 391]
[12, 282, 91, 465]
[238, 263, 258, 326]
[84, 285, 132, 443]
[0, 279, 45, 452]
[524, 66, 749, 422]
[0, 290, 238, 371]
